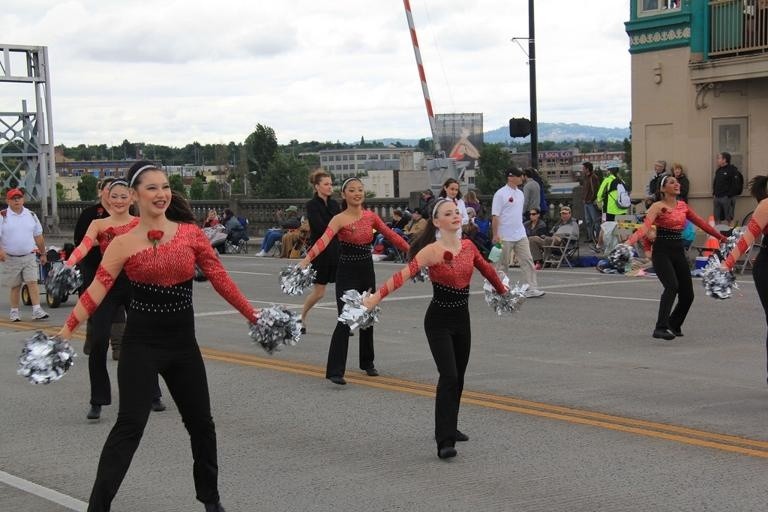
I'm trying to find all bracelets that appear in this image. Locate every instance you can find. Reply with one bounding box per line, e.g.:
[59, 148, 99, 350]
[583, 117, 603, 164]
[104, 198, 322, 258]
[39, 252, 47, 256]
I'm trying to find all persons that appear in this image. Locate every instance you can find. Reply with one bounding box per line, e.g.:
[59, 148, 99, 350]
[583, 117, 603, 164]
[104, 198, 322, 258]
[201, 206, 247, 255]
[361, 196, 508, 457]
[483, 166, 544, 300]
[258, 206, 310, 258]
[59, 160, 258, 512]
[74, 175, 128, 360]
[296, 169, 342, 334]
[723, 175, 768, 384]
[712, 151, 743, 228]
[59, 181, 166, 419]
[579, 162, 690, 271]
[372, 168, 579, 270]
[622, 173, 729, 340]
[0, 189, 49, 321]
[291, 177, 409, 385]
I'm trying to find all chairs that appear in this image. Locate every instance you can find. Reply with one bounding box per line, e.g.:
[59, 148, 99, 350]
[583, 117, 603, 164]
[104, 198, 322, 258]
[271, 239, 282, 257]
[391, 242, 406, 263]
[226, 217, 248, 256]
[735, 241, 755, 275]
[692, 247, 718, 258]
[541, 212, 580, 270]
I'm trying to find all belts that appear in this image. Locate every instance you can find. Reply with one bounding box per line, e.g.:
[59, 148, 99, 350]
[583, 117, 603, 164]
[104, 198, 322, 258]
[7, 253, 27, 258]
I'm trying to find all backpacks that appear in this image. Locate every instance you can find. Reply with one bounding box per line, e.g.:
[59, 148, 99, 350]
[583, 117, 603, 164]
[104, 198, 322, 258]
[608, 178, 631, 208]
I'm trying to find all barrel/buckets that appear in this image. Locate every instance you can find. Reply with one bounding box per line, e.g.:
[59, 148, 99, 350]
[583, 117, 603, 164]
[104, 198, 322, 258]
[695, 255, 709, 278]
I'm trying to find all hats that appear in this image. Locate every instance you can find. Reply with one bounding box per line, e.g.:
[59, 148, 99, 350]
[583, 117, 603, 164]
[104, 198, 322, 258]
[412, 208, 421, 214]
[7, 189, 23, 200]
[286, 206, 298, 212]
[506, 168, 522, 177]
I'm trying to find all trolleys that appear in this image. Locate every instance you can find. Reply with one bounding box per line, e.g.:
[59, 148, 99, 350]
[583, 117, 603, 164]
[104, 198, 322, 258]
[21, 244, 82, 308]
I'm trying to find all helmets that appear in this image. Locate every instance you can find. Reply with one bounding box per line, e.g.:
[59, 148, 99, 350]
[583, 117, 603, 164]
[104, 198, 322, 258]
[607, 161, 619, 168]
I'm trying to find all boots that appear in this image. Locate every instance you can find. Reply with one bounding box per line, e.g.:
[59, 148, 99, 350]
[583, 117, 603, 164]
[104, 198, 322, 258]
[110, 323, 125, 359]
[83, 319, 92, 355]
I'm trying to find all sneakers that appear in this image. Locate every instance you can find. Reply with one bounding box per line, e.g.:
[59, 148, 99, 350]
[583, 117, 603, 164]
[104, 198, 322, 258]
[87, 404, 101, 419]
[439, 429, 469, 459]
[301, 324, 307, 335]
[328, 374, 346, 387]
[368, 367, 378, 374]
[203, 502, 224, 512]
[31, 309, 47, 321]
[9, 310, 20, 322]
[150, 399, 165, 410]
[255, 249, 271, 257]
[653, 322, 683, 339]
[526, 289, 545, 297]
